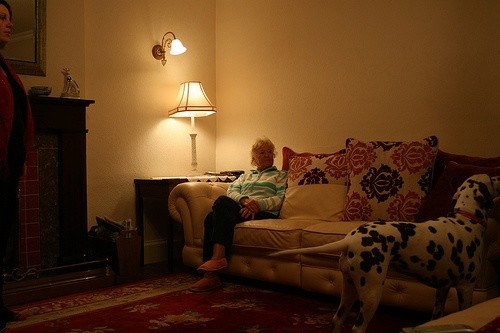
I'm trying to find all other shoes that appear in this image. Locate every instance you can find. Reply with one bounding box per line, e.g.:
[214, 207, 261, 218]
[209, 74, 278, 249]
[190, 277, 222, 291]
[197, 258, 228, 271]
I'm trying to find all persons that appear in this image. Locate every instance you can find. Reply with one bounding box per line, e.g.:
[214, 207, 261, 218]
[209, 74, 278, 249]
[191, 139, 287, 291]
[0, 0, 33, 333]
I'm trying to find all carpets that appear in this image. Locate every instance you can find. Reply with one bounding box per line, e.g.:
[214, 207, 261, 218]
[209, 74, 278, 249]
[0, 267, 420, 333]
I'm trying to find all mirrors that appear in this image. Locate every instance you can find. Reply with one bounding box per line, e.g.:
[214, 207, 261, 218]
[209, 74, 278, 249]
[1, 0, 47, 77]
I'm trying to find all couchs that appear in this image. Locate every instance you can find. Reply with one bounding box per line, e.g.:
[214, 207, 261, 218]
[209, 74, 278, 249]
[166, 132, 500, 319]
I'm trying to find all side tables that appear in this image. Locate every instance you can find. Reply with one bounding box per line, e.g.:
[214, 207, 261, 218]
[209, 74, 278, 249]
[133, 174, 238, 277]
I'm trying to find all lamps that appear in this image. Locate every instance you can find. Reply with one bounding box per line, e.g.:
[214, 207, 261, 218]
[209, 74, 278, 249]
[152, 31, 187, 67]
[167, 80, 217, 177]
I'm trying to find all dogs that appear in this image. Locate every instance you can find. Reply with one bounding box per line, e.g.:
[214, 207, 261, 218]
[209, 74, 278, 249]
[268, 173, 500, 333]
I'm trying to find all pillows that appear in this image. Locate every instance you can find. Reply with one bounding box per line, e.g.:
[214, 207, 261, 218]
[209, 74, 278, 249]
[341, 135, 440, 223]
[277, 149, 353, 222]
[416, 160, 500, 224]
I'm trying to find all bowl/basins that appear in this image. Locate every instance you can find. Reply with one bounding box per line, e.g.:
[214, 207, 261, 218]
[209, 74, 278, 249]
[31, 86, 52, 96]
